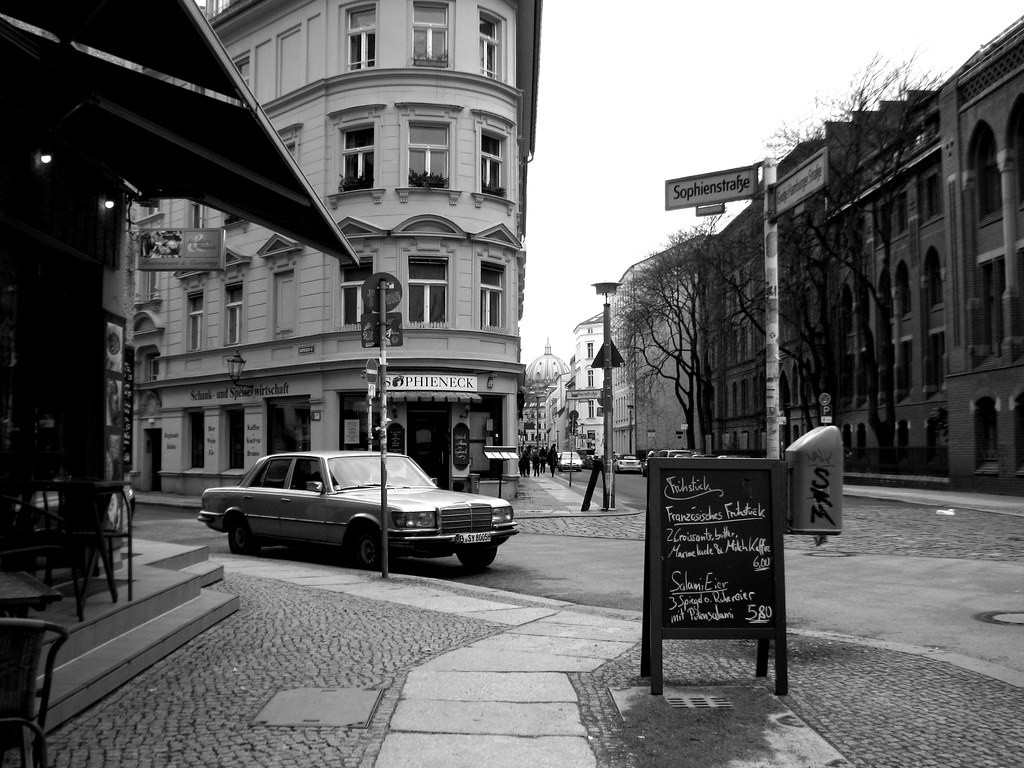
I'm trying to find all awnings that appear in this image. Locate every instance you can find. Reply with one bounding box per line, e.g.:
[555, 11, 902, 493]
[0, 0, 360, 267]
[378, 391, 482, 404]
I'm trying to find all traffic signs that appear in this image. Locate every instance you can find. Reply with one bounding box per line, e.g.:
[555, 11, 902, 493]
[665, 160, 762, 217]
[767, 146, 828, 225]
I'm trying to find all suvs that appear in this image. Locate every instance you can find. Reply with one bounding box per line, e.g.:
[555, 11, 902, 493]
[643, 450, 692, 477]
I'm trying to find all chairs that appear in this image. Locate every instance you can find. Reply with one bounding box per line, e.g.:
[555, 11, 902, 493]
[0, 619, 68, 768]
[0, 491, 84, 622]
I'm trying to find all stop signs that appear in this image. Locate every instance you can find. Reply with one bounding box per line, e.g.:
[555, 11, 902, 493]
[366, 358, 378, 384]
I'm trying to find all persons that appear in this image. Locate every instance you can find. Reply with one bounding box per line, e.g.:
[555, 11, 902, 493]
[518, 450, 533, 477]
[539, 448, 547, 473]
[533, 455, 540, 477]
[547, 446, 558, 477]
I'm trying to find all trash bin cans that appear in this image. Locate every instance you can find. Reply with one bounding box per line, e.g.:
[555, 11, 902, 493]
[785, 425, 843, 534]
[468, 473, 480, 494]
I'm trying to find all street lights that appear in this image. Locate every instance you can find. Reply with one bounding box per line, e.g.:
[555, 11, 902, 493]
[591, 282, 622, 511]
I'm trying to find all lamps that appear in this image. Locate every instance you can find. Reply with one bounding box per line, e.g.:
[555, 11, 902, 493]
[228, 349, 252, 389]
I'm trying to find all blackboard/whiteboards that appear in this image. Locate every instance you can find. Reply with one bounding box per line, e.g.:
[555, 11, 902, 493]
[641, 459, 785, 639]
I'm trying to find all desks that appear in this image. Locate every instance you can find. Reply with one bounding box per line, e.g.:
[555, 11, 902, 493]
[38, 480, 137, 603]
[0, 568, 62, 618]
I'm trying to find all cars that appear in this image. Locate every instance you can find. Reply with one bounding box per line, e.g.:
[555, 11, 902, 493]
[557, 452, 583, 472]
[197, 451, 519, 571]
[613, 454, 642, 474]
[580, 455, 604, 469]
